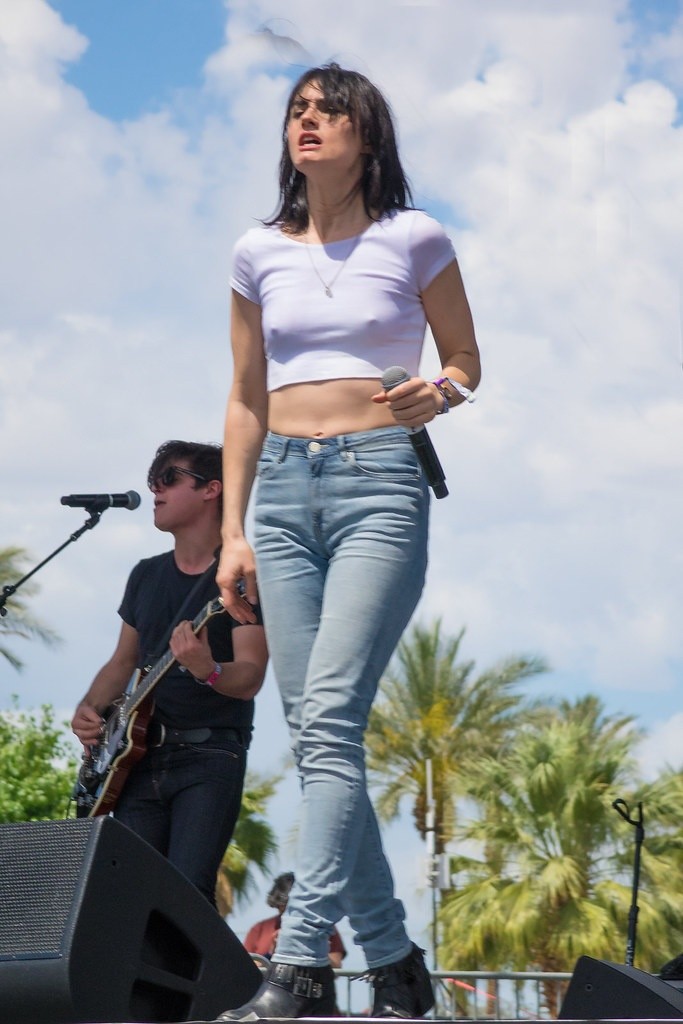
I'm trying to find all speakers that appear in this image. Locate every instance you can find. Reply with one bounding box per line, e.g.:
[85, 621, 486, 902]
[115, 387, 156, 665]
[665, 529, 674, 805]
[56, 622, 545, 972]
[0, 815, 265, 1024]
[557, 955, 683, 1020]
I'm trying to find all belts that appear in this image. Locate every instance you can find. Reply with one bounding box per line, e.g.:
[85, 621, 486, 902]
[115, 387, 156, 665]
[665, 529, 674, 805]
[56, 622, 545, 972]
[145, 728, 250, 747]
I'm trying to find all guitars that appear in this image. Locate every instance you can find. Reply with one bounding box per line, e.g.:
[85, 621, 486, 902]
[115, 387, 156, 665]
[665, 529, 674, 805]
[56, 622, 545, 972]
[75, 581, 249, 818]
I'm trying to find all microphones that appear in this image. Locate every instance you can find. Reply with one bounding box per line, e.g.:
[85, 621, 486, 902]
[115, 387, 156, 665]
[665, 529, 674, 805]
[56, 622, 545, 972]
[61, 490, 140, 511]
[381, 367, 448, 499]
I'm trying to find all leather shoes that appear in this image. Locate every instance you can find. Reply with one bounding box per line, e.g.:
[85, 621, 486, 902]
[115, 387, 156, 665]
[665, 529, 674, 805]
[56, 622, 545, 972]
[214, 962, 337, 1020]
[350, 942, 435, 1018]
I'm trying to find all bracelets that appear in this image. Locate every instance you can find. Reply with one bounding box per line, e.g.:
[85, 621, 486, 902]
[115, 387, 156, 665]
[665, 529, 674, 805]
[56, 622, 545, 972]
[195, 664, 222, 685]
[429, 376, 477, 413]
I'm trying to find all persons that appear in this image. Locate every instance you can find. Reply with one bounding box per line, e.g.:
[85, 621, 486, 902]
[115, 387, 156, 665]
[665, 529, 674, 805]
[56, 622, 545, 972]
[215, 58, 481, 1023]
[72, 440, 269, 902]
[243, 872, 347, 980]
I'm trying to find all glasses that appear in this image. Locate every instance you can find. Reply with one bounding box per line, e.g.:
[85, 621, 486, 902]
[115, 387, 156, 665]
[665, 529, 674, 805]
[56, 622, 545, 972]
[147, 466, 207, 489]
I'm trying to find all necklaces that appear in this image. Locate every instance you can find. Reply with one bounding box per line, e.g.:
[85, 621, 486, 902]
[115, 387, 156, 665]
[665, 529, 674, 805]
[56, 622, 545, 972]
[301, 227, 362, 296]
